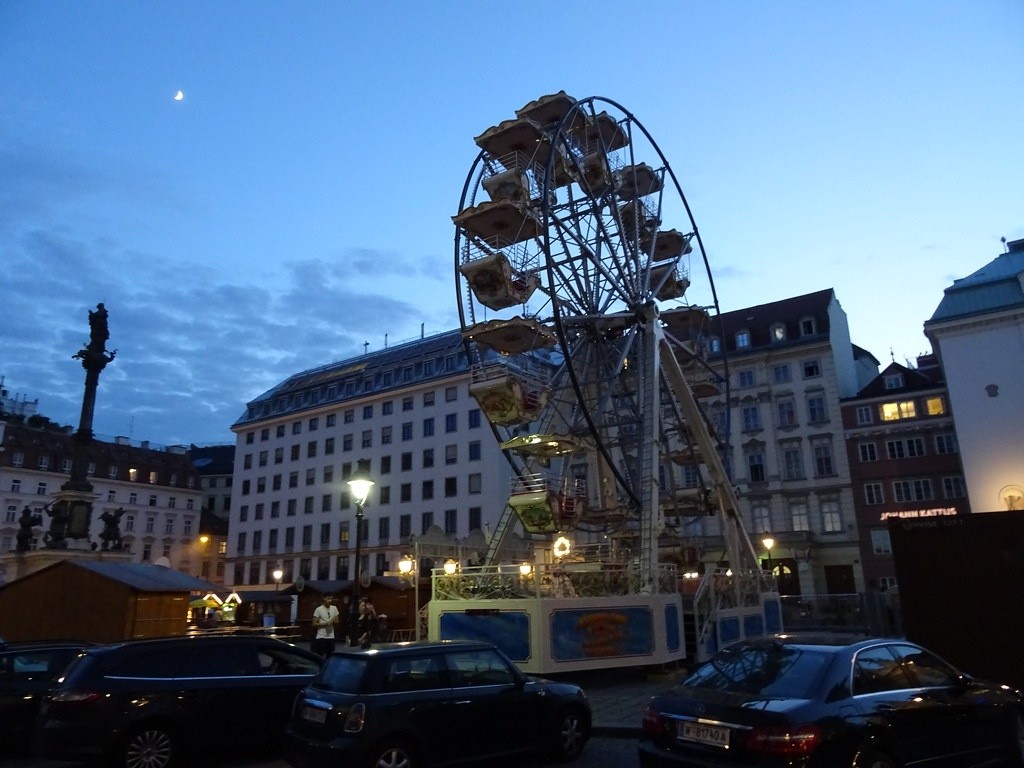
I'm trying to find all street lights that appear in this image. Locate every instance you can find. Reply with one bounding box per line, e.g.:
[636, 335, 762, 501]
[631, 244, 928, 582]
[272, 568, 284, 596]
[399, 539, 422, 640]
[763, 530, 775, 572]
[348, 454, 374, 646]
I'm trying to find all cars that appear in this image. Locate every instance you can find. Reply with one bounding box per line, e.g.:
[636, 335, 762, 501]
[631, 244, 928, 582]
[635, 629, 1024, 768]
[36, 631, 324, 768]
[0, 638, 123, 768]
[288, 637, 593, 768]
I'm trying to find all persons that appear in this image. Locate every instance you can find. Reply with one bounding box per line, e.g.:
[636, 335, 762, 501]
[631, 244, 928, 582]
[313, 592, 339, 659]
[345, 599, 371, 650]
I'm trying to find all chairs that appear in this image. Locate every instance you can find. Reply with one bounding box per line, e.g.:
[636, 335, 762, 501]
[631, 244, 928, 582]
[392, 660, 414, 684]
[426, 656, 460, 688]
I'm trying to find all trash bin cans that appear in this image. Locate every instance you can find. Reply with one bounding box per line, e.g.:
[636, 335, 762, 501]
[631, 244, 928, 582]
[263, 614, 275, 633]
